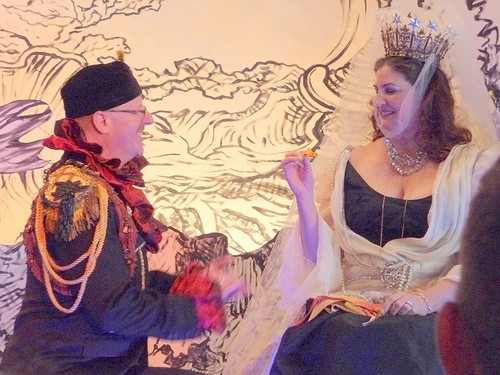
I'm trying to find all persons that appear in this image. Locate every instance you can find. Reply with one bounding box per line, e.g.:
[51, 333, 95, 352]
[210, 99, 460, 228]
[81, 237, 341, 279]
[221, 6, 500, 375]
[436, 155, 500, 375]
[0, 61, 248, 375]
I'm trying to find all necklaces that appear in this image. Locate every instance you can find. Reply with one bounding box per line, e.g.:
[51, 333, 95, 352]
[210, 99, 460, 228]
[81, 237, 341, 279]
[385, 136, 428, 176]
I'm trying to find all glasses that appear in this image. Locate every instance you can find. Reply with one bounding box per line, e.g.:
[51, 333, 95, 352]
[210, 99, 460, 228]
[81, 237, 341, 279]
[108, 106, 146, 118]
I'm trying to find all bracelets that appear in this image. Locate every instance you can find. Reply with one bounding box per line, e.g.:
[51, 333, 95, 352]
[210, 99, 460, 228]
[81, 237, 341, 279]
[417, 292, 432, 315]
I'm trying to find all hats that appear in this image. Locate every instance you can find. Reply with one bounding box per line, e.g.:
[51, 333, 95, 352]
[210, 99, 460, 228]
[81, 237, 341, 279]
[60, 61, 143, 118]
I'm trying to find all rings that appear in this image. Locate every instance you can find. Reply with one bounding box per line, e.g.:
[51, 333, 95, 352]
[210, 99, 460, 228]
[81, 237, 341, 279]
[404, 301, 413, 306]
